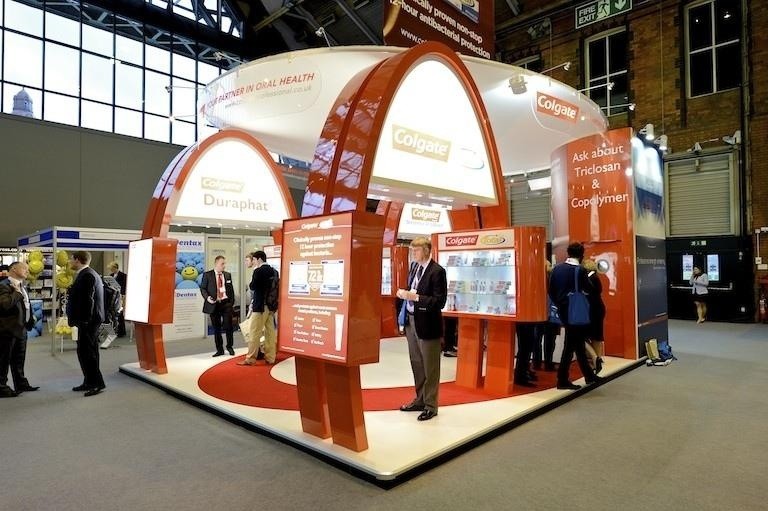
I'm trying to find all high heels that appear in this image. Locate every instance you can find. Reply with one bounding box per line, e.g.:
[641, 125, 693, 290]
[596, 356, 602, 374]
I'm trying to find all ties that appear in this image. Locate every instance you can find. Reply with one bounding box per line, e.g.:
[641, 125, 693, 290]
[217, 274, 223, 298]
[409, 266, 422, 305]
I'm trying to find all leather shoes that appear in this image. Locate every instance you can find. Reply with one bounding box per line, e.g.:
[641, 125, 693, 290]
[400, 403, 423, 410]
[239, 361, 256, 365]
[527, 365, 554, 387]
[0, 387, 35, 397]
[557, 382, 581, 388]
[585, 375, 605, 384]
[213, 346, 232, 355]
[73, 383, 105, 396]
[418, 410, 437, 419]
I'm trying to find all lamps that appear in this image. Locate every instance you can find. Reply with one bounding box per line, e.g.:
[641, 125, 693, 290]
[600, 101, 638, 115]
[212, 50, 245, 70]
[542, 61, 573, 76]
[164, 84, 206, 96]
[314, 26, 333, 47]
[578, 82, 615, 95]
[636, 123, 669, 151]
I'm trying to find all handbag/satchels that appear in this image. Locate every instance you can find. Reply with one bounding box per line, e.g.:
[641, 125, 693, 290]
[547, 293, 562, 324]
[644, 339, 673, 365]
[239, 318, 265, 342]
[567, 293, 590, 324]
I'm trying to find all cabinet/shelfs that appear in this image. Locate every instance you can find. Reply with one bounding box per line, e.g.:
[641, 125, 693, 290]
[18, 247, 59, 323]
[431, 226, 548, 324]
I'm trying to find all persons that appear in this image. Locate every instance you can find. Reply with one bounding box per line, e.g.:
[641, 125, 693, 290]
[0, 262, 40, 398]
[201, 256, 234, 356]
[245, 253, 276, 360]
[438, 314, 488, 357]
[67, 251, 106, 396]
[689, 264, 709, 323]
[236, 251, 279, 366]
[395, 237, 447, 420]
[107, 261, 127, 337]
[515, 242, 606, 389]
